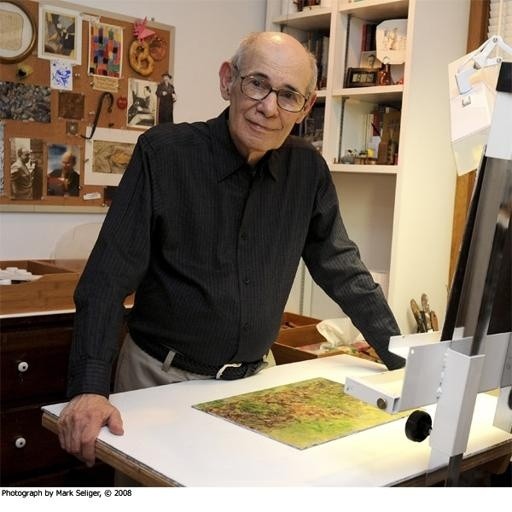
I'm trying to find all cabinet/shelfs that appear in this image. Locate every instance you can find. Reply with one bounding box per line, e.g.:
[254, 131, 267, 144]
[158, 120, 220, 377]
[264, 1, 471, 334]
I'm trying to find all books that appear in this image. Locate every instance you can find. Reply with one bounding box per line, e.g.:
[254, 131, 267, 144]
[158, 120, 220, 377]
[365, 24, 385, 71]
[365, 101, 401, 165]
[298, 0, 321, 13]
[362, 23, 371, 50]
[294, 29, 328, 89]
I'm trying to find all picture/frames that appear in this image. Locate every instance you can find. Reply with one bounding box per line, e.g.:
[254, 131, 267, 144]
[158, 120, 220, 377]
[38, 4, 83, 66]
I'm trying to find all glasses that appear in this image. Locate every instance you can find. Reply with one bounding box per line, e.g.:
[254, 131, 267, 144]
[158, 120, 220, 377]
[231, 64, 312, 113]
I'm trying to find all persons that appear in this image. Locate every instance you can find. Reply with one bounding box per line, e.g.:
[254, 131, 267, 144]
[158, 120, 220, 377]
[9, 145, 45, 197]
[47, 152, 79, 196]
[130, 81, 157, 123]
[154, 71, 176, 123]
[382, 25, 402, 52]
[364, 52, 376, 72]
[58, 31, 411, 471]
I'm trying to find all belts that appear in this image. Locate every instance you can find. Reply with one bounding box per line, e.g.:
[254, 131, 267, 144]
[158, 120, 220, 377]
[126, 326, 268, 380]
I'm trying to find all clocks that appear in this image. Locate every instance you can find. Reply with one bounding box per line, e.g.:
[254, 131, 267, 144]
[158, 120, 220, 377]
[1, 1, 38, 65]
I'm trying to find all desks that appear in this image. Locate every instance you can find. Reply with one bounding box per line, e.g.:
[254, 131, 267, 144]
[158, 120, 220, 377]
[39, 329, 511, 486]
